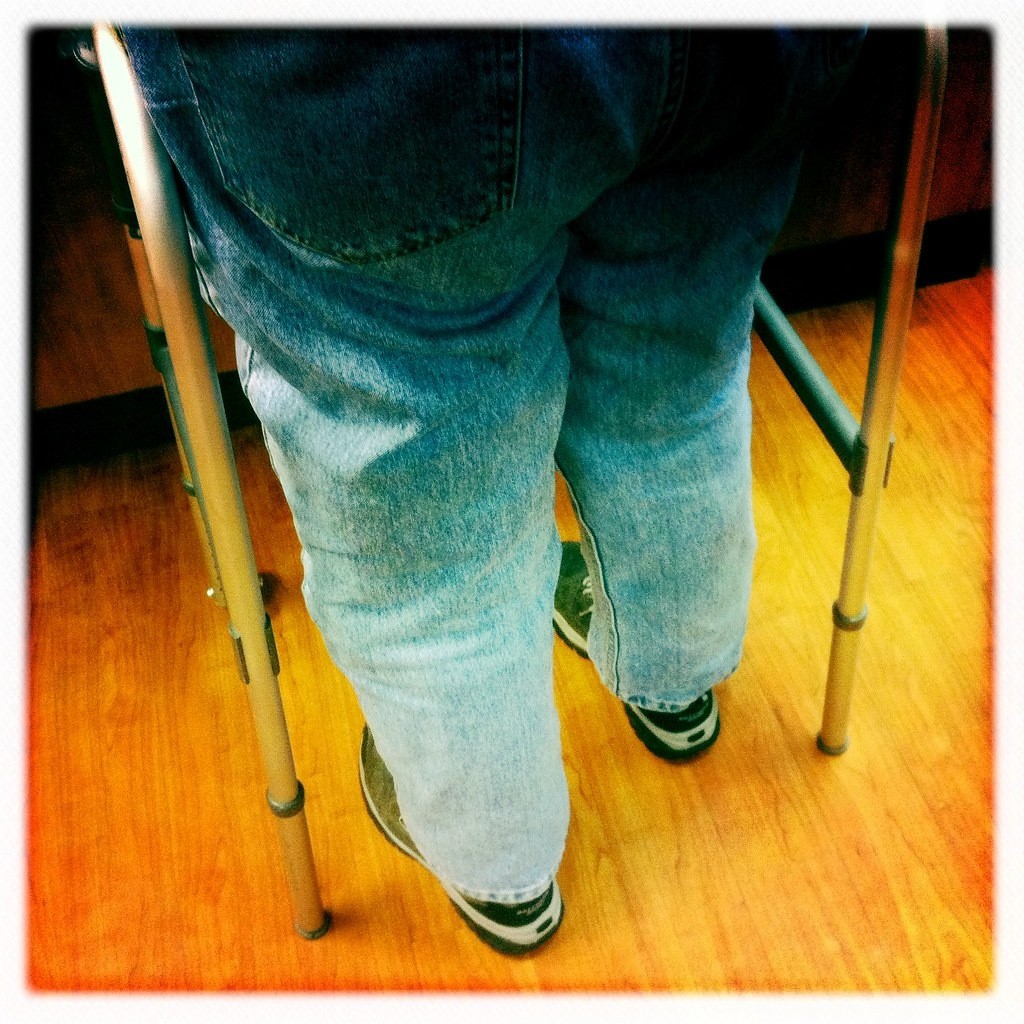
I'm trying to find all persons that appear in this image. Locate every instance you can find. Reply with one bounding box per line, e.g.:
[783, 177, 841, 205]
[76, 27, 870, 954]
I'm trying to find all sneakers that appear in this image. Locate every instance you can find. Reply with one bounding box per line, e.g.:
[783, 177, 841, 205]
[552, 542, 719, 758]
[359, 722, 564, 954]
